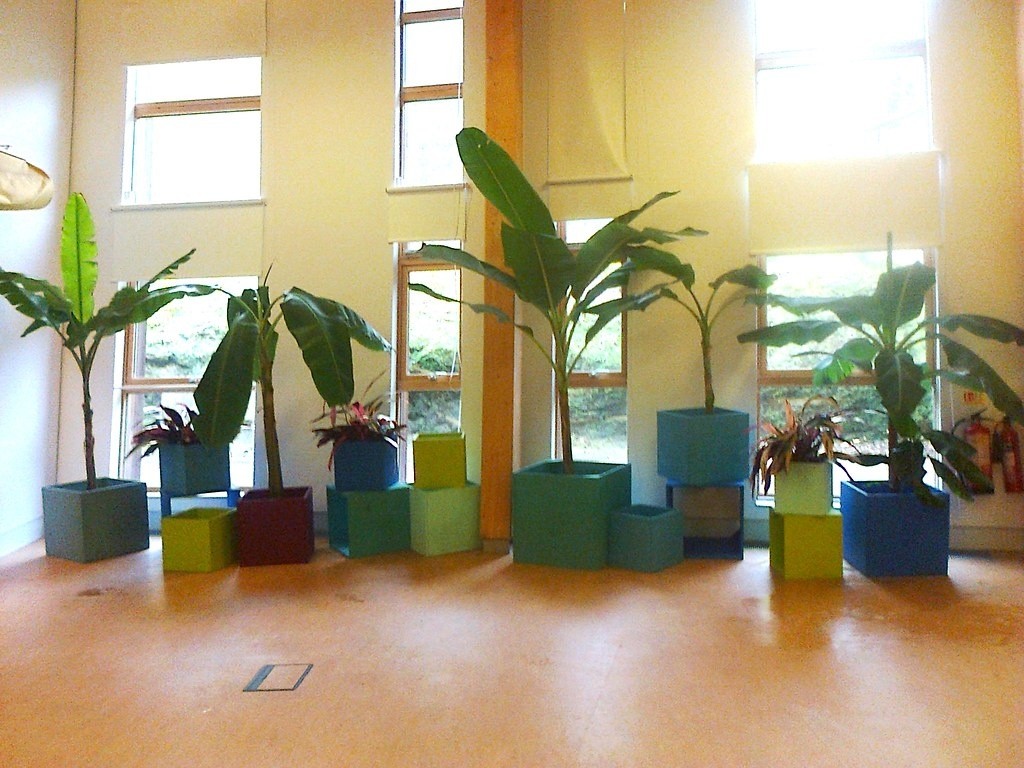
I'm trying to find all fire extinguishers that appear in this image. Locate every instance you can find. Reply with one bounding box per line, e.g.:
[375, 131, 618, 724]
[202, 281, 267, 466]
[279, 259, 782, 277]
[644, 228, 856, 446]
[991, 415, 1024, 494]
[951, 405, 994, 492]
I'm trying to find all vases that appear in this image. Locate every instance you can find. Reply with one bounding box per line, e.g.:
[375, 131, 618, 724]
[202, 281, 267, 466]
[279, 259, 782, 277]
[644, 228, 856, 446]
[327, 482, 411, 557]
[161, 507, 237, 573]
[769, 507, 844, 577]
[608, 504, 683, 573]
[409, 480, 482, 554]
[414, 432, 468, 488]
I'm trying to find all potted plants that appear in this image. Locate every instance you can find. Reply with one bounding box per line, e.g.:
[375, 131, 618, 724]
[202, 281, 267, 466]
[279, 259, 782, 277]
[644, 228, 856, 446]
[93, 257, 397, 567]
[738, 232, 1024, 578]
[407, 126, 708, 572]
[124, 403, 231, 495]
[743, 394, 861, 513]
[0, 193, 194, 563]
[313, 366, 408, 491]
[615, 259, 791, 486]
